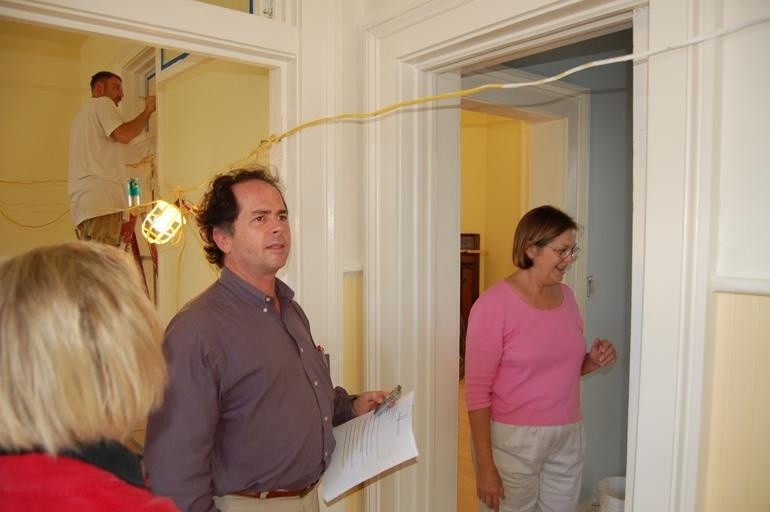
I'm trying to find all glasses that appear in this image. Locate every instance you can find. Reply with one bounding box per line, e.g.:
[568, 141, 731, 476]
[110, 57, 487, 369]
[540, 243, 582, 259]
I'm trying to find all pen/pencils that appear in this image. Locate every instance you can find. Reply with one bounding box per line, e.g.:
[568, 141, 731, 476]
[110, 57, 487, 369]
[139, 96, 147, 98]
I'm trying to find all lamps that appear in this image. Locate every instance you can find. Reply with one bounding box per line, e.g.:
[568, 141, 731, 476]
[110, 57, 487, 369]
[141, 194, 189, 245]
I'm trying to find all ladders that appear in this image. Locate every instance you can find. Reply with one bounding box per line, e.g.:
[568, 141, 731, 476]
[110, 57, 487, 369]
[117, 207, 158, 310]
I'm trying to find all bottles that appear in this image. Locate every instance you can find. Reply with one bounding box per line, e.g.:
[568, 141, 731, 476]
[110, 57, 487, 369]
[127, 175, 140, 207]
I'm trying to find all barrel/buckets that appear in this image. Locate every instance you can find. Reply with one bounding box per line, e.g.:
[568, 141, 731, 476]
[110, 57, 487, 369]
[591, 476, 625, 511]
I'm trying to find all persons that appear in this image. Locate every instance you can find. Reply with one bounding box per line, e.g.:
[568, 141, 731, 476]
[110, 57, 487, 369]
[461, 204, 620, 511]
[136, 162, 399, 511]
[1, 240, 187, 512]
[67, 71, 157, 253]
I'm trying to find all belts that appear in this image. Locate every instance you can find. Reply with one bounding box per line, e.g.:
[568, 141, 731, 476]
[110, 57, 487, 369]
[230, 480, 318, 500]
